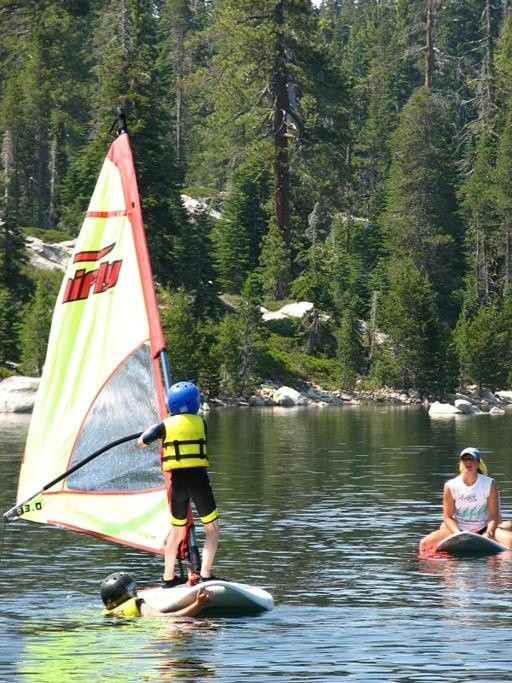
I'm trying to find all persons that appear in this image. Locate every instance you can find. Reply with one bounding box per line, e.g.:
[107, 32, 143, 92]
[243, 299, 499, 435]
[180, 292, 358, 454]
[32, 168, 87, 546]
[101, 569, 216, 622]
[136, 380, 229, 588]
[418, 446, 512, 552]
[440, 458, 512, 532]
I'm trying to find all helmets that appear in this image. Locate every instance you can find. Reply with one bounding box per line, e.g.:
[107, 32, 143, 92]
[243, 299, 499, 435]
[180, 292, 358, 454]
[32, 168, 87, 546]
[99, 571, 138, 611]
[166, 380, 201, 414]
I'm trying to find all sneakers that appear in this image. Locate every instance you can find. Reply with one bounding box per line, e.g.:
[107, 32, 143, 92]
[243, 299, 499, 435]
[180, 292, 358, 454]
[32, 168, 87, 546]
[161, 575, 189, 588]
[199, 574, 228, 582]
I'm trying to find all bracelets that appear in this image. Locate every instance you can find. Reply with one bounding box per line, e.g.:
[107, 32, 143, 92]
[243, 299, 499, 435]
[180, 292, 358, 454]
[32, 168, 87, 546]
[484, 530, 494, 537]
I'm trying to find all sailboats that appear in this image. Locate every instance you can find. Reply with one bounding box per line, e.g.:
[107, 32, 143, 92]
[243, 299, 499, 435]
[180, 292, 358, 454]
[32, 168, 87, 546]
[3, 106, 273, 612]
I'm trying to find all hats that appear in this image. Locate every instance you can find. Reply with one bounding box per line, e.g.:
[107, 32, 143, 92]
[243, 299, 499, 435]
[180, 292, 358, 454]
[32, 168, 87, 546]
[460, 446, 480, 461]
